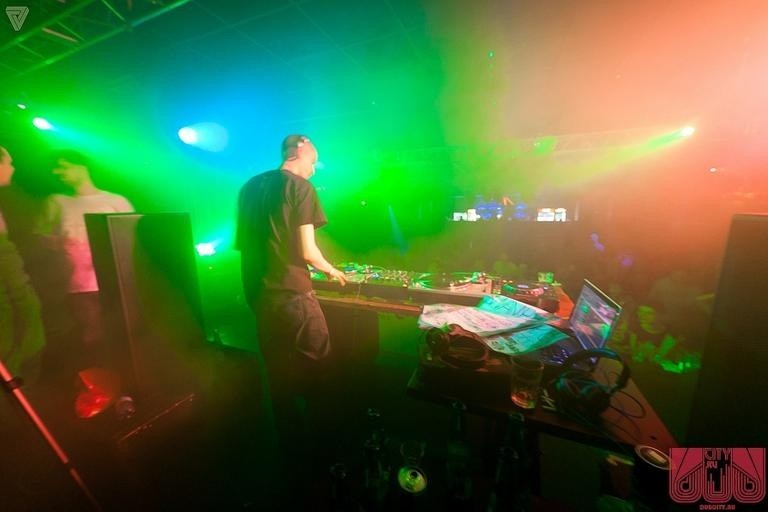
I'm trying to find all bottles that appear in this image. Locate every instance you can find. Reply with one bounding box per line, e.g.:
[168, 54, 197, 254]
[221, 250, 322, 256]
[327, 398, 533, 511]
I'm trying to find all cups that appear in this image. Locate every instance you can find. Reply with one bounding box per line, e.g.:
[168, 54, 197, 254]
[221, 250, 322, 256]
[509, 354, 545, 411]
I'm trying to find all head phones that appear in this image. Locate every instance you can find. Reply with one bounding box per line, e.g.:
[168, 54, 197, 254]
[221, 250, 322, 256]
[283, 135, 309, 160]
[426, 326, 489, 369]
[553, 347, 630, 418]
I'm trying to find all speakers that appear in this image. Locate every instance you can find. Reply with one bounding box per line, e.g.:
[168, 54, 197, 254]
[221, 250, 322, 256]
[686, 213, 768, 448]
[83, 212, 213, 403]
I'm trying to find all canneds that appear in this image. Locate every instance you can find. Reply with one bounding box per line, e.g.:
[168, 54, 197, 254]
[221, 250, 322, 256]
[390, 465, 429, 504]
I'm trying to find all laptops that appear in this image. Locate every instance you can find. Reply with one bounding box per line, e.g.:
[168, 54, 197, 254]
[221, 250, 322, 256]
[537, 278, 623, 373]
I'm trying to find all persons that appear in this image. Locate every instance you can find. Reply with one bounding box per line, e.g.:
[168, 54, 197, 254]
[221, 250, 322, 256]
[233, 134, 355, 459]
[28, 149, 138, 346]
[1, 146, 46, 380]
